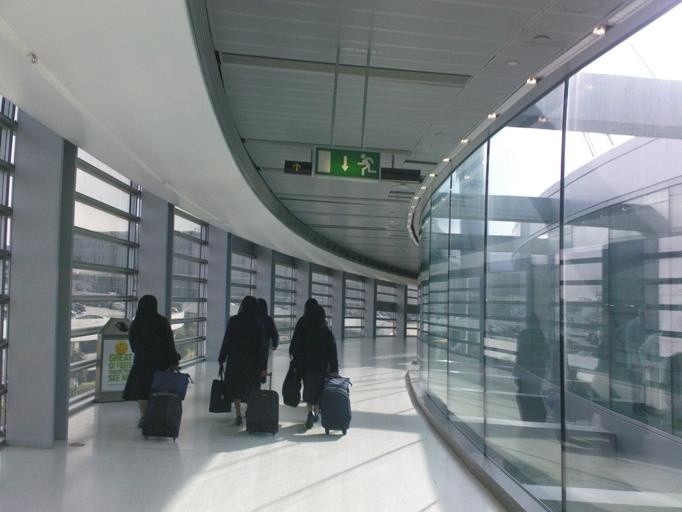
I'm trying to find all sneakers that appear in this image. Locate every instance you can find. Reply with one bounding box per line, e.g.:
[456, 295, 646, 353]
[236, 415, 243, 425]
[306, 410, 319, 430]
[138, 415, 145, 428]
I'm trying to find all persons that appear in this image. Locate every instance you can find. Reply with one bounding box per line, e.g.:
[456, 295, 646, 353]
[218, 295, 268, 425]
[296, 299, 330, 409]
[257, 299, 279, 384]
[512, 303, 661, 415]
[292, 305, 339, 428]
[122, 294, 179, 428]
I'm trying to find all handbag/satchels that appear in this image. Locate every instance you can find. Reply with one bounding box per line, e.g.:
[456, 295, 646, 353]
[209, 366, 232, 413]
[150, 367, 193, 399]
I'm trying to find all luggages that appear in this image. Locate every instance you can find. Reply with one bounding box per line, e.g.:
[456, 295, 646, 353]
[245, 370, 281, 438]
[141, 364, 195, 441]
[319, 374, 353, 436]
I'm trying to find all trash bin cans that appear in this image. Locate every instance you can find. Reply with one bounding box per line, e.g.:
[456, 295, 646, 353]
[92, 318, 135, 403]
